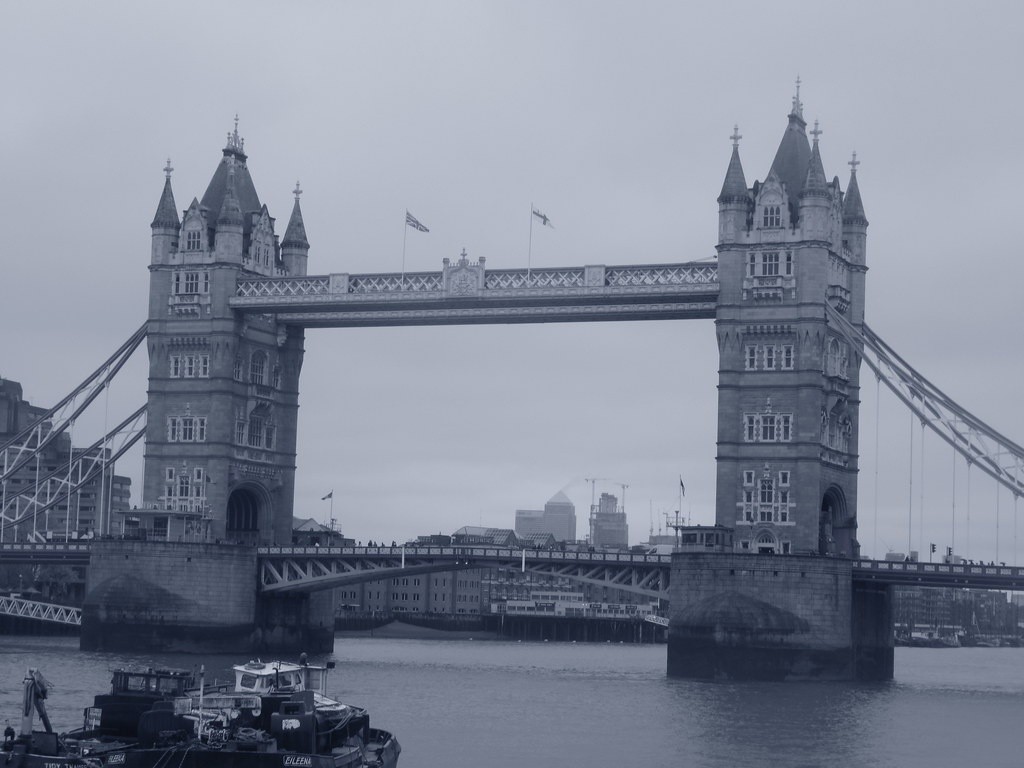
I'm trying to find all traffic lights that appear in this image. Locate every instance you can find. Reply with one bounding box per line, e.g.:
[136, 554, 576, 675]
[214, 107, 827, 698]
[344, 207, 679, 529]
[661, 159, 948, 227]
[949, 547, 952, 556]
[932, 544, 936, 552]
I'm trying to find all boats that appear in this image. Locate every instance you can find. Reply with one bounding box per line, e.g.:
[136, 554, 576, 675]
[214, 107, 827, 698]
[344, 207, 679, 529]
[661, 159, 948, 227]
[0, 652, 402, 768]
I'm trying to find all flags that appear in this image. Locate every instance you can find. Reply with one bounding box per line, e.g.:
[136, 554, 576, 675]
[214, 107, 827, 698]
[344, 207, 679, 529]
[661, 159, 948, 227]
[406, 212, 429, 233]
[321, 492, 332, 500]
[680, 479, 685, 496]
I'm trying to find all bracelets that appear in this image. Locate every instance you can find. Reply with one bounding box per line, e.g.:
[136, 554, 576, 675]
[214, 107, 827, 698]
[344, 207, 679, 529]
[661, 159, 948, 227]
[531, 205, 553, 228]
